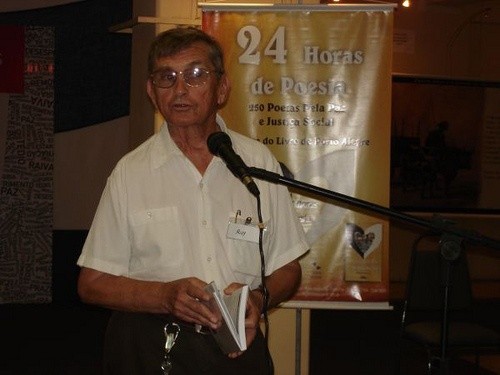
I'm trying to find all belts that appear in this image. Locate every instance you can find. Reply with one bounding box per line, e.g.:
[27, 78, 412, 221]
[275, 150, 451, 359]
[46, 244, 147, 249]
[149, 312, 212, 336]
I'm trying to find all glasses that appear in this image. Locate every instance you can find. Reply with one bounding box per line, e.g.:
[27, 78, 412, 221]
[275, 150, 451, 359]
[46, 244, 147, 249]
[149, 66, 224, 89]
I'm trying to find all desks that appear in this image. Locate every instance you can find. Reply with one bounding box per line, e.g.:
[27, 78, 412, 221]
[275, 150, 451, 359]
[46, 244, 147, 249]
[256, 297, 395, 375]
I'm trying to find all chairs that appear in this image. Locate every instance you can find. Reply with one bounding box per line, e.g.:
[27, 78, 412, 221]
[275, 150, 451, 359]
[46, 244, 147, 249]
[399, 232, 500, 375]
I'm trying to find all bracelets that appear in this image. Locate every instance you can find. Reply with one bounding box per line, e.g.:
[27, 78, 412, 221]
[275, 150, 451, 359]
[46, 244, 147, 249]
[252, 285, 271, 312]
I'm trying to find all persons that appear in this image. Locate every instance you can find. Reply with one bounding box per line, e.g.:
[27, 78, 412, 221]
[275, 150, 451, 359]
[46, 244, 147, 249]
[75, 26, 313, 375]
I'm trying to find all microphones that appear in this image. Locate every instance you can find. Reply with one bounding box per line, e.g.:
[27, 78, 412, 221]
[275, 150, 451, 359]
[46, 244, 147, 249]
[207, 132, 261, 198]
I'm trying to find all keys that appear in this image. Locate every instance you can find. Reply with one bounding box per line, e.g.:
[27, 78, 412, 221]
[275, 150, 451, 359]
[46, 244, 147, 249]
[160, 351, 173, 374]
